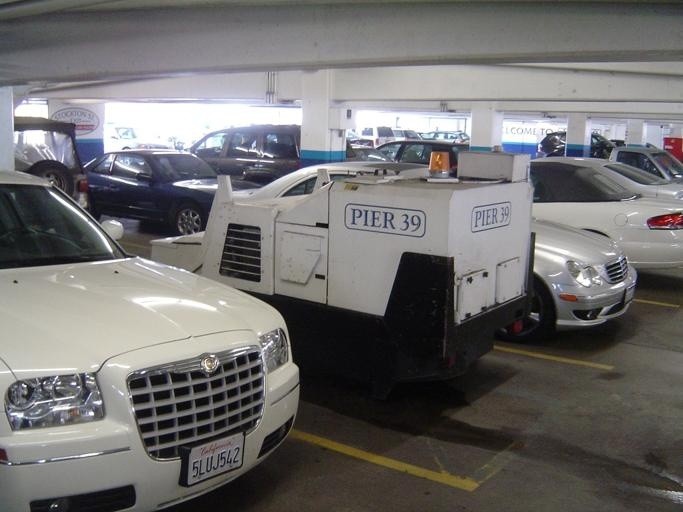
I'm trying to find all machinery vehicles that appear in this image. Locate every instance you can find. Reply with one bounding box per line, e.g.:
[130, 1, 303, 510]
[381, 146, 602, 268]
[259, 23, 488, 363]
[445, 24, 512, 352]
[148, 147, 538, 419]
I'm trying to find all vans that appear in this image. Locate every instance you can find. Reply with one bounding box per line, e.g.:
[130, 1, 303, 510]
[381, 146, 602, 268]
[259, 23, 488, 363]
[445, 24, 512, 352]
[345, 124, 471, 162]
[189, 124, 364, 187]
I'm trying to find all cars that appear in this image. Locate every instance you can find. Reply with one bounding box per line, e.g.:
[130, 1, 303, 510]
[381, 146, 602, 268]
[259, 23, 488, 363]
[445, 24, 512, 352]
[80, 147, 266, 237]
[503, 129, 683, 344]
[0, 169, 301, 512]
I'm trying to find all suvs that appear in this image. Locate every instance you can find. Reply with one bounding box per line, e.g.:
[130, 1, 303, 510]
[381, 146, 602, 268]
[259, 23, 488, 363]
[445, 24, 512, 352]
[13, 118, 97, 236]
